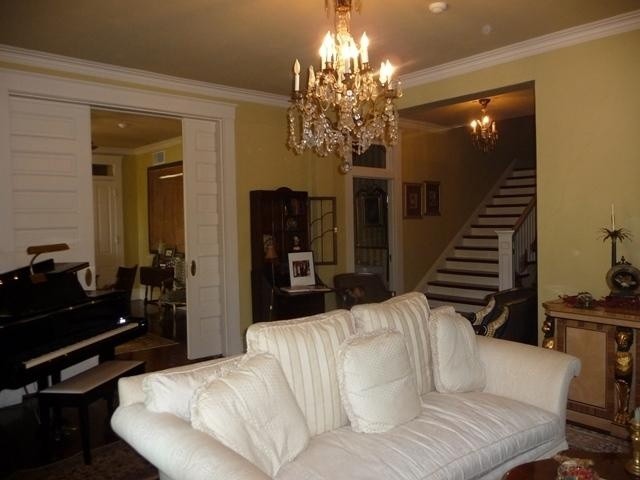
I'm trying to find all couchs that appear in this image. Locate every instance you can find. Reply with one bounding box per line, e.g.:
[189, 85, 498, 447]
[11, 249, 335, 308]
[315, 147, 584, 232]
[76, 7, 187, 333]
[110, 333, 582, 480]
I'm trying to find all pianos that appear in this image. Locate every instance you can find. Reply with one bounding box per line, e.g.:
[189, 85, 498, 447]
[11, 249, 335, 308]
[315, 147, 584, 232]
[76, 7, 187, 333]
[0, 257, 153, 392]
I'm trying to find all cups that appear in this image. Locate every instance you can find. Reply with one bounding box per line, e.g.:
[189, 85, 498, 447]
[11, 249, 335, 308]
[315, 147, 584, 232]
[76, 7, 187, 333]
[558, 459, 595, 480]
[576, 293, 592, 310]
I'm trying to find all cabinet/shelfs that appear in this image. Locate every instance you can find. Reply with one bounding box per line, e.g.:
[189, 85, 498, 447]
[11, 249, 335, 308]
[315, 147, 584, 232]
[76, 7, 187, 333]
[541, 295, 640, 441]
[249, 186, 339, 324]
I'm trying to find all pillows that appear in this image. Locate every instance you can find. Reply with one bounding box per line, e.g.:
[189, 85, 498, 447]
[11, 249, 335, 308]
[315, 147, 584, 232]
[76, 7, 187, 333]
[189, 349, 313, 478]
[427, 308, 487, 395]
[245, 308, 359, 440]
[350, 290, 436, 397]
[335, 328, 425, 435]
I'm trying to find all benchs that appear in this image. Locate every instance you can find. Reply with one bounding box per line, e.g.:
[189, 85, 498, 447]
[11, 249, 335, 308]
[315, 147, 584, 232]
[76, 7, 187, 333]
[37, 359, 148, 462]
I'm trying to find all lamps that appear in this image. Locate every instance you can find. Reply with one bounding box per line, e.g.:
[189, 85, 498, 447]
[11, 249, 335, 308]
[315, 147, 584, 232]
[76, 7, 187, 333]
[469, 98, 504, 158]
[285, 2, 404, 175]
[26, 243, 70, 277]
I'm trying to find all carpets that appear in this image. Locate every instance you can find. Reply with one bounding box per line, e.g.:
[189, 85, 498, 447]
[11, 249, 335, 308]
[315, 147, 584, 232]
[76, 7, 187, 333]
[114, 333, 180, 355]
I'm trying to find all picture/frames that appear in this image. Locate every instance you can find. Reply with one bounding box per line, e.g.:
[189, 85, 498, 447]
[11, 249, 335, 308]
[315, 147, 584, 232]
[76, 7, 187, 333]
[403, 182, 424, 219]
[287, 250, 317, 288]
[423, 180, 442, 217]
[162, 246, 175, 259]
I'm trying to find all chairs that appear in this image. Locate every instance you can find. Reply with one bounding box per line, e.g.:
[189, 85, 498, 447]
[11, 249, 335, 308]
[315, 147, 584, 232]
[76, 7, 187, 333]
[100, 264, 138, 299]
